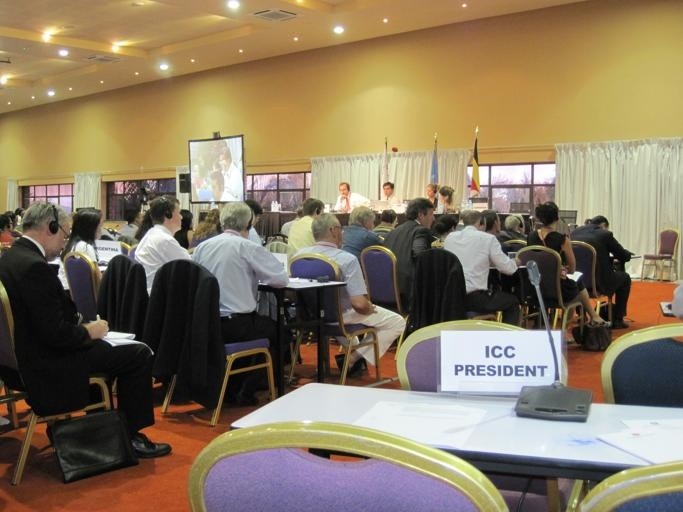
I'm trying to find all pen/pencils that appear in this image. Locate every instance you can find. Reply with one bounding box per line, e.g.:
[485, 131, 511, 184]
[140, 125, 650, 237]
[96, 314, 100, 321]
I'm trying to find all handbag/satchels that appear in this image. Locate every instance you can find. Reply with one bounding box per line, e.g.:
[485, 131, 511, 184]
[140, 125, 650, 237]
[571, 325, 610, 351]
[46, 410, 140, 484]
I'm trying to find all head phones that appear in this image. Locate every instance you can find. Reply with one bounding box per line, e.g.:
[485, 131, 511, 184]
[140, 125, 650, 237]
[49, 204, 58, 233]
[161, 196, 173, 219]
[5, 216, 11, 228]
[316, 200, 322, 214]
[247, 208, 253, 230]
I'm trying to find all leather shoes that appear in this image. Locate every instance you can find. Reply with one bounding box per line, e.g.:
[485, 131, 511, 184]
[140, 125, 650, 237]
[335, 354, 361, 378]
[235, 392, 258, 407]
[613, 321, 628, 329]
[131, 432, 171, 459]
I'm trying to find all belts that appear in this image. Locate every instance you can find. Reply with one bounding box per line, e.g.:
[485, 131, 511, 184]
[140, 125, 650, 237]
[221, 312, 255, 321]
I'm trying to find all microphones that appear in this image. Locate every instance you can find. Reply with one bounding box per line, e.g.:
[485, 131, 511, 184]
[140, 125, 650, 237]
[514, 261, 593, 423]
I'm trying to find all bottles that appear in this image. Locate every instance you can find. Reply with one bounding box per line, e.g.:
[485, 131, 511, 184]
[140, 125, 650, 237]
[270, 200, 279, 212]
[460, 199, 473, 210]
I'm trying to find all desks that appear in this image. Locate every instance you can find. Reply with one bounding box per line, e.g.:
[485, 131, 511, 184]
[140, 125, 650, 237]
[229, 382, 683, 511]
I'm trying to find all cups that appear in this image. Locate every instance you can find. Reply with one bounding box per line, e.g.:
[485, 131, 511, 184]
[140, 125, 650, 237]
[324, 203, 330, 213]
[507, 252, 517, 259]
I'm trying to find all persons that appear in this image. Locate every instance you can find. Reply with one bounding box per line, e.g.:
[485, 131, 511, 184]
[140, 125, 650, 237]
[190, 140, 243, 202]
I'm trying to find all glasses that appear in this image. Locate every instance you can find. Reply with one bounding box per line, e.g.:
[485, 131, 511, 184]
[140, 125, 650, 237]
[59, 224, 71, 240]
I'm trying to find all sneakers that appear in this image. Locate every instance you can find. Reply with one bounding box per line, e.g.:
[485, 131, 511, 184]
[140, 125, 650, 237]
[591, 317, 613, 328]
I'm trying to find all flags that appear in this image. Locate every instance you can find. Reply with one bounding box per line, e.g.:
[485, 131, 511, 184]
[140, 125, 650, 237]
[469, 137, 480, 198]
[382, 142, 388, 185]
[429, 140, 439, 200]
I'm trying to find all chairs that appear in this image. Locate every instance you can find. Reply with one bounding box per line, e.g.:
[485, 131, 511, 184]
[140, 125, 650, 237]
[396, 320, 569, 511]
[600, 323, 683, 408]
[361, 241, 611, 360]
[0, 237, 381, 485]
[188, 421, 509, 511]
[641, 229, 680, 283]
[569, 461, 682, 511]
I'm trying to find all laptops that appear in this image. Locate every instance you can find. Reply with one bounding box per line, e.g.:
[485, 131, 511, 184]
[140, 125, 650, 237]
[370, 199, 390, 211]
